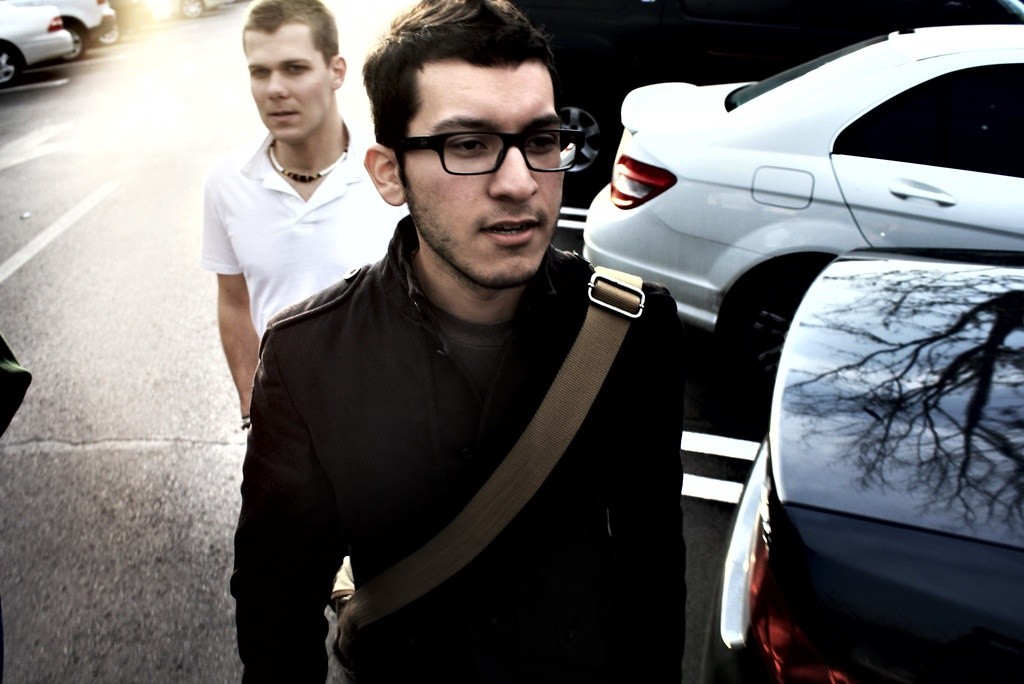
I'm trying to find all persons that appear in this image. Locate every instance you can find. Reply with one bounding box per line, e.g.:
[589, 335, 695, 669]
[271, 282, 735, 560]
[230, 1, 697, 684]
[203, 0, 412, 656]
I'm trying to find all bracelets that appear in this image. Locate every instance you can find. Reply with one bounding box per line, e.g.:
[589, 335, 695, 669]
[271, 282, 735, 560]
[241, 415, 251, 430]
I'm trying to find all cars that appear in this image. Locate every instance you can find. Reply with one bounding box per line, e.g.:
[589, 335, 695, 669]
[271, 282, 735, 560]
[507, 0, 1024, 219]
[580, 23, 1024, 409]
[703, 246, 1024, 683]
[0, 0, 235, 88]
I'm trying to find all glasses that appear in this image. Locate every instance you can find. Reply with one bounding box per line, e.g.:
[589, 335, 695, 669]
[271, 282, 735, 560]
[399, 124, 585, 175]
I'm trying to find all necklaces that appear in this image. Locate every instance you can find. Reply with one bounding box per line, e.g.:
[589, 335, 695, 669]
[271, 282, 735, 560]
[268, 138, 349, 184]
